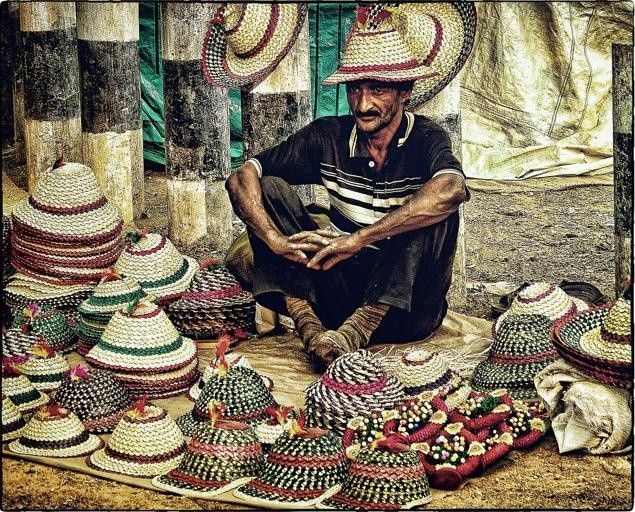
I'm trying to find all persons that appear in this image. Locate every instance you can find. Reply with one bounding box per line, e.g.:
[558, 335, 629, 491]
[225, 67, 473, 378]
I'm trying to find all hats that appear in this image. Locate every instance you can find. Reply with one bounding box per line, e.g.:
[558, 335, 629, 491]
[349, 2, 476, 110]
[321, 29, 440, 85]
[202, 3, 306, 88]
[275, 283, 631, 509]
[1, 164, 276, 509]
